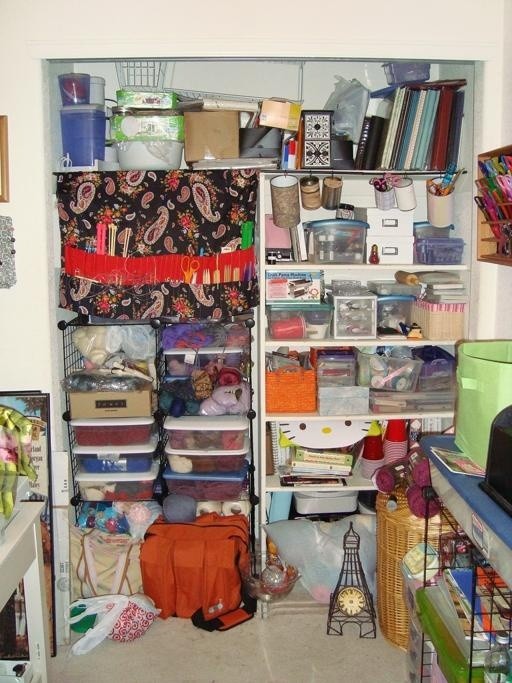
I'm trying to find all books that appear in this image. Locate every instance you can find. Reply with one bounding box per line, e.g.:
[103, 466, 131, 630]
[426, 282, 468, 304]
[279, 448, 353, 486]
[354, 79, 466, 171]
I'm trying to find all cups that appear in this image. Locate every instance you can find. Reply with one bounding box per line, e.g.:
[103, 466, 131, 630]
[270, 175, 301, 227]
[393, 177, 417, 210]
[307, 323, 328, 339]
[427, 177, 456, 228]
[61, 107, 105, 166]
[320, 176, 343, 209]
[58, 73, 89, 106]
[362, 434, 384, 479]
[299, 175, 322, 210]
[270, 316, 306, 339]
[384, 418, 408, 464]
[374, 178, 395, 209]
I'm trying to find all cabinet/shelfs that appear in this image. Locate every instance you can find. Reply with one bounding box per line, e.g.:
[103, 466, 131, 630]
[39, 56, 486, 619]
[411, 489, 511, 682]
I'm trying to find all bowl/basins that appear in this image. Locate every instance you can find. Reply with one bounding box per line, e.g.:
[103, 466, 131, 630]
[114, 140, 184, 170]
[240, 553, 302, 600]
[313, 225, 366, 262]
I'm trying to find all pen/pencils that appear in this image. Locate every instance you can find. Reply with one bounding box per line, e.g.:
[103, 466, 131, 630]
[430, 162, 462, 196]
[474, 190, 509, 223]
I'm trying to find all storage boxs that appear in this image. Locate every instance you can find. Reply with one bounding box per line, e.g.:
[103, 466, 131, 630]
[310, 354, 359, 386]
[324, 290, 376, 342]
[308, 216, 371, 265]
[412, 347, 453, 390]
[353, 203, 416, 267]
[264, 351, 316, 416]
[373, 295, 417, 341]
[182, 108, 241, 163]
[71, 343, 252, 504]
[409, 300, 466, 341]
[293, 490, 363, 516]
[451, 336, 511, 480]
[269, 303, 336, 342]
[414, 237, 466, 267]
[357, 351, 421, 395]
[369, 280, 423, 300]
[314, 384, 373, 417]
[415, 430, 511, 594]
[265, 269, 329, 304]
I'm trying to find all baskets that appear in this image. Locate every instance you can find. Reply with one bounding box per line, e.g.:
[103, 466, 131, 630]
[265, 365, 318, 413]
[115, 62, 168, 94]
[375, 487, 457, 651]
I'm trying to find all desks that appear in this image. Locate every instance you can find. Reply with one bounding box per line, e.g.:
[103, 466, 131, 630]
[2, 502, 58, 683]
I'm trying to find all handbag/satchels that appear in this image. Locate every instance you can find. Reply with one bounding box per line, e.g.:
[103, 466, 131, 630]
[138, 511, 256, 632]
[69, 523, 140, 600]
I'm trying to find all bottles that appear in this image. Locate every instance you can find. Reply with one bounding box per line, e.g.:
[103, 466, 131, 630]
[483, 631, 511, 682]
[367, 244, 381, 265]
[89, 75, 107, 105]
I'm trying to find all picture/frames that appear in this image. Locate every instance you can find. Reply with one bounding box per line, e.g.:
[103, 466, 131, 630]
[1, 391, 58, 662]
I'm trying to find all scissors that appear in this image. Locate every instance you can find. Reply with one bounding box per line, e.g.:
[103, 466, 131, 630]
[369, 173, 402, 191]
[475, 150, 512, 198]
